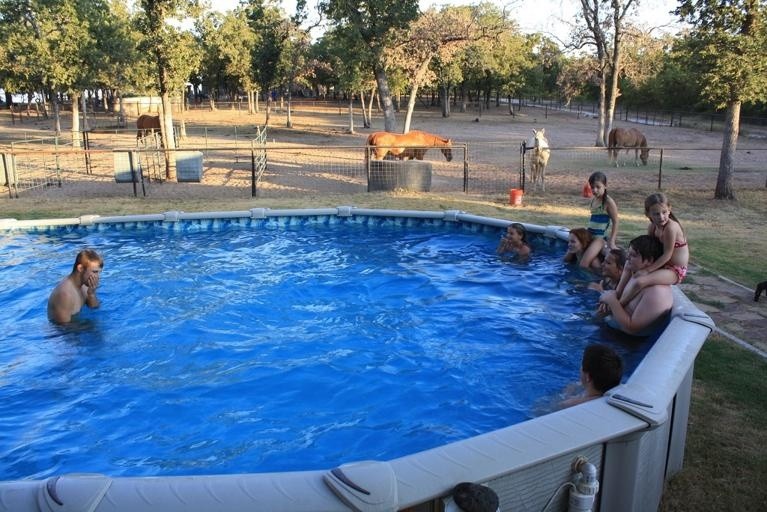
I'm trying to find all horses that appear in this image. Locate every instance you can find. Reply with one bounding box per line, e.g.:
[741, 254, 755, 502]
[607, 126, 651, 168]
[136, 114, 163, 148]
[364, 129, 455, 166]
[527, 127, 551, 193]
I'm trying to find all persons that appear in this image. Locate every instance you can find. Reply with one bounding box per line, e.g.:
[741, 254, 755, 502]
[588, 249, 625, 293]
[595, 193, 689, 315]
[567, 227, 603, 269]
[559, 342, 623, 408]
[564, 172, 621, 268]
[47, 250, 103, 331]
[596, 235, 675, 338]
[498, 222, 529, 256]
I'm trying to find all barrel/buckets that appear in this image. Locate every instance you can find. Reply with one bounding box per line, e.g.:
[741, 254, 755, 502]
[510, 188, 524, 205]
[583, 184, 593, 197]
